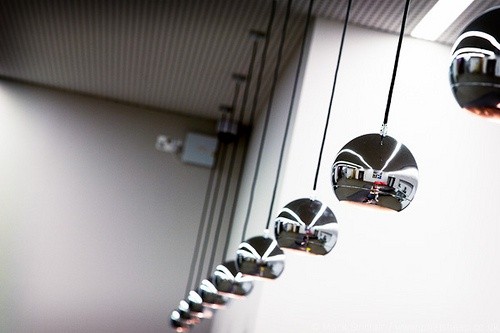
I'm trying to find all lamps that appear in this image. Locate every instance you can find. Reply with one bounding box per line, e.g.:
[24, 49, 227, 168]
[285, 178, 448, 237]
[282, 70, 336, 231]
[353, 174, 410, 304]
[167, 0, 499, 333]
